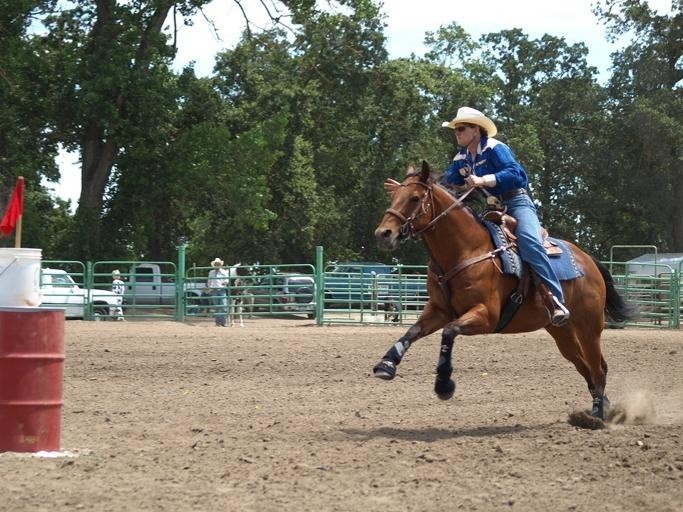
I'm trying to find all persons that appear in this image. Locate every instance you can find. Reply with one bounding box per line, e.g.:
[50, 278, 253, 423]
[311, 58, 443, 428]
[111, 270, 125, 321]
[442, 106, 572, 327]
[207, 257, 229, 327]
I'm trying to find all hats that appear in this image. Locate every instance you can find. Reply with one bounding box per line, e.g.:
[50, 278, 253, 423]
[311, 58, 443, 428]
[441, 107, 498, 138]
[210, 258, 225, 267]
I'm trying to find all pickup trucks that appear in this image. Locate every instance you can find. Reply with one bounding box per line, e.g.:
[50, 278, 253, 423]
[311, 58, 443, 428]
[124, 264, 208, 313]
[324, 262, 428, 309]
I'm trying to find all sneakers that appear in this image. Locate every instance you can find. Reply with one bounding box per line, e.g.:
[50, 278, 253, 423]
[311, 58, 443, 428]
[551, 309, 568, 327]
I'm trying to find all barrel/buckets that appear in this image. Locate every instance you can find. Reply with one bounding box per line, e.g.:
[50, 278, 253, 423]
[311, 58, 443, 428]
[0, 247, 42, 306]
[0, 307, 67, 453]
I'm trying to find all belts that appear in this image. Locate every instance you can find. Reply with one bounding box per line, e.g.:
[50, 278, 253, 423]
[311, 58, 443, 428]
[486, 189, 524, 206]
[217, 289, 221, 291]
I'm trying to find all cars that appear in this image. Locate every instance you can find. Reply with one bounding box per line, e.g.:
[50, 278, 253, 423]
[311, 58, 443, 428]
[41, 270, 119, 322]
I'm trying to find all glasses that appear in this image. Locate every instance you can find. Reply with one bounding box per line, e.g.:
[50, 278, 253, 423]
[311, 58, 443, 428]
[454, 126, 470, 132]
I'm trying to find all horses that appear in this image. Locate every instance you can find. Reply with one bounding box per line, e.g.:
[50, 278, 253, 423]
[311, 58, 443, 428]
[371, 158, 641, 422]
[226, 264, 261, 328]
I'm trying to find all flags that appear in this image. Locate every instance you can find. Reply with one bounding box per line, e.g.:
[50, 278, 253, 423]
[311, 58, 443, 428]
[0, 178, 24, 235]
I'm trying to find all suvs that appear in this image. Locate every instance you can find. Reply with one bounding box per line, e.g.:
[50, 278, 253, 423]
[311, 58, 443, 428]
[242, 273, 317, 318]
